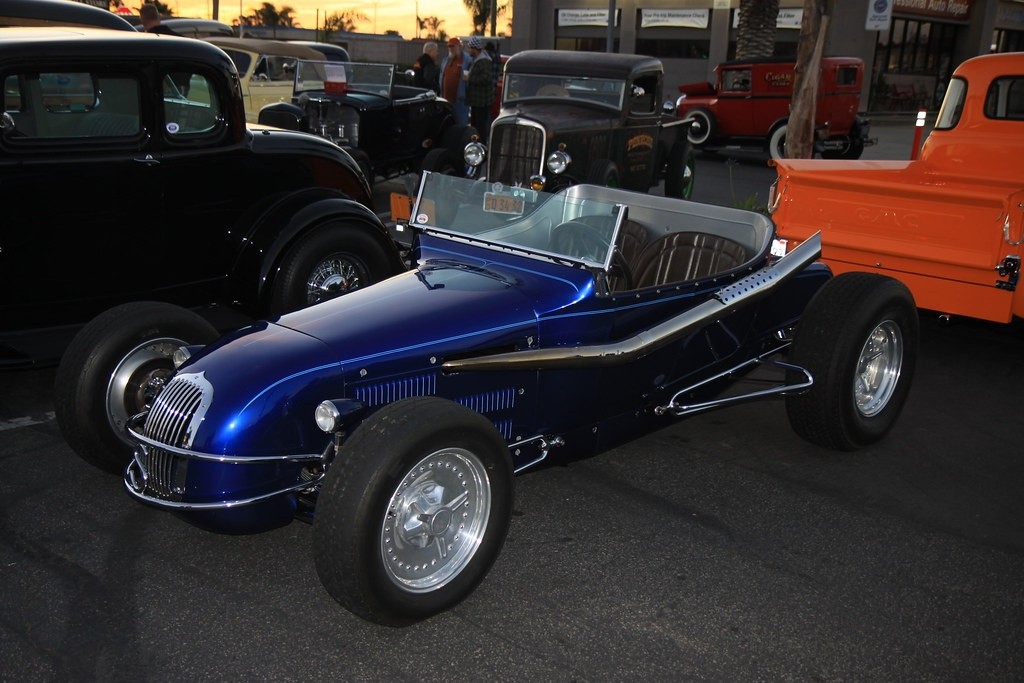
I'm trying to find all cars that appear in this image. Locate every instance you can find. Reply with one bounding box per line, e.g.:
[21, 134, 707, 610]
[53, 169, 922, 630]
[0, 1, 481, 203]
[461, 48, 695, 204]
[0, 24, 374, 421]
[676, 57, 877, 171]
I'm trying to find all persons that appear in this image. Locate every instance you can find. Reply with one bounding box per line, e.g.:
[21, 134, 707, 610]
[462, 38, 494, 145]
[439, 38, 471, 125]
[141, 5, 192, 99]
[413, 42, 440, 96]
[484, 41, 500, 97]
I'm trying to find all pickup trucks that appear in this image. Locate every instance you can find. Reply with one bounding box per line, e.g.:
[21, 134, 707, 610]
[766, 51, 1023, 353]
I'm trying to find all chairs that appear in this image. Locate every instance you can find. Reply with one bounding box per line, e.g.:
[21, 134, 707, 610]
[543, 214, 651, 297]
[888, 82, 930, 111]
[612, 230, 749, 293]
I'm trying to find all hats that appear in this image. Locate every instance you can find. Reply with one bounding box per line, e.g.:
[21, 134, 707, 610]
[467, 36, 482, 50]
[447, 37, 464, 47]
